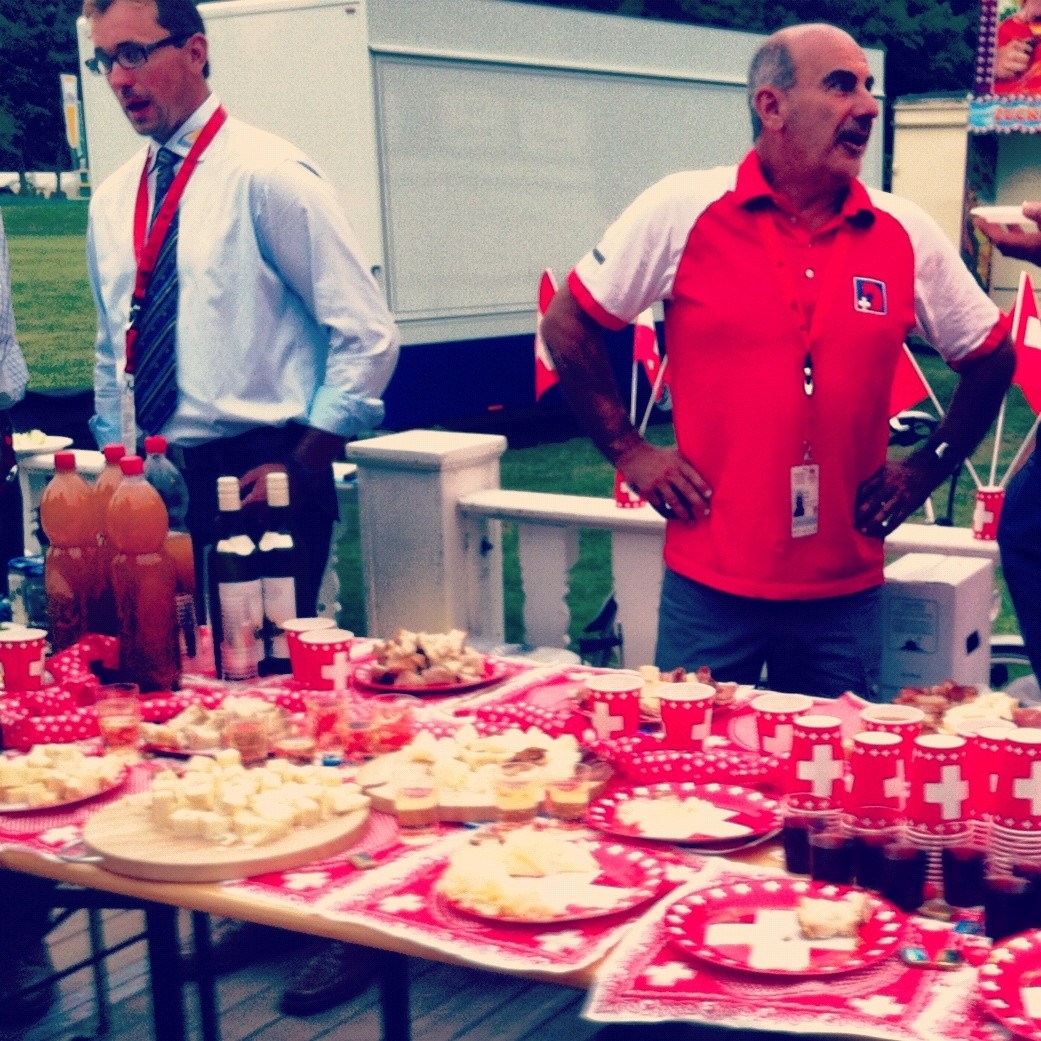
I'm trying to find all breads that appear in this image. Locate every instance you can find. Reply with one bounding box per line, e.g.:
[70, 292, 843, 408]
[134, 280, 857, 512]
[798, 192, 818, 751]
[639, 665, 739, 716]
[142, 695, 285, 751]
[370, 629, 485, 686]
[895, 681, 1021, 732]
[796, 892, 873, 938]
[150, 750, 371, 847]
[403, 724, 581, 792]
[0, 743, 124, 806]
[471, 827, 598, 918]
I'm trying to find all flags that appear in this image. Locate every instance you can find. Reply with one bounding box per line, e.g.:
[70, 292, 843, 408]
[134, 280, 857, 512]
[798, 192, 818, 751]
[535, 273, 557, 399]
[888, 342, 929, 416]
[1010, 273, 1041, 415]
[633, 308, 663, 400]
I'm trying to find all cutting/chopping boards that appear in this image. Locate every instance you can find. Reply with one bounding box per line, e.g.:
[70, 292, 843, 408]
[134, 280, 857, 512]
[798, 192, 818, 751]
[60, 788, 370, 880]
[357, 746, 545, 822]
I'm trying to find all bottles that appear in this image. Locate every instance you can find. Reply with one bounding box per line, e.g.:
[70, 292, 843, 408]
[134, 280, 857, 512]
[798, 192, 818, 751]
[41, 436, 178, 691]
[206, 476, 269, 680]
[141, 437, 194, 594]
[255, 472, 301, 674]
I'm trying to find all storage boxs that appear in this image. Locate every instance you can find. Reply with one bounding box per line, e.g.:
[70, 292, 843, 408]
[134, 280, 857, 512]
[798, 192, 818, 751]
[883, 552, 994, 699]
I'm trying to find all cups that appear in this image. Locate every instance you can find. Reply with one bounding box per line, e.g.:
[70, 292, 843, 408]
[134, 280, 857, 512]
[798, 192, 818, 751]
[584, 673, 715, 749]
[220, 596, 258, 683]
[0, 628, 47, 691]
[493, 763, 540, 827]
[391, 780, 440, 847]
[225, 694, 418, 765]
[283, 617, 353, 690]
[543, 763, 592, 828]
[93, 683, 141, 750]
[752, 690, 1041, 944]
[175, 594, 204, 674]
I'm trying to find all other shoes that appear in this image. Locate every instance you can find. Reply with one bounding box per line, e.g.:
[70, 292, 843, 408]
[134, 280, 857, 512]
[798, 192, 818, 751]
[20, 940, 53, 1009]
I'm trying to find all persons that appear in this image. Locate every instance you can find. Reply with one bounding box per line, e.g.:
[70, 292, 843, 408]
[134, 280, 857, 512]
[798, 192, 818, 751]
[541, 20, 1017, 702]
[84, 1, 402, 617]
[973, 196, 1041, 690]
[994, 0, 1041, 97]
[0, 214, 31, 589]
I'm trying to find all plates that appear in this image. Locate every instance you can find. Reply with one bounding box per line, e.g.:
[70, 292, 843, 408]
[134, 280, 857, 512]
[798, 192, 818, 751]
[977, 930, 1041, 1041]
[586, 781, 786, 844]
[970, 206, 1033, 224]
[566, 696, 660, 724]
[12, 435, 74, 454]
[0, 752, 133, 814]
[437, 841, 662, 924]
[665, 879, 907, 977]
[355, 658, 507, 692]
[138, 743, 280, 762]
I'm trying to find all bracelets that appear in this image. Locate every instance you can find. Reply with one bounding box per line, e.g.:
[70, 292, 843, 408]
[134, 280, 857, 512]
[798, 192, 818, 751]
[924, 438, 961, 476]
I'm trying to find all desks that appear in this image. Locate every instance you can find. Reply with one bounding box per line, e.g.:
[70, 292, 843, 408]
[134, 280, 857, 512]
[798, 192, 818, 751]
[0, 634, 1041, 1041]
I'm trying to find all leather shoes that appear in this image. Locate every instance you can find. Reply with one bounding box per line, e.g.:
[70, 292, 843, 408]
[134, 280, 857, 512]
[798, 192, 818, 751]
[181, 918, 257, 968]
[280, 941, 380, 1017]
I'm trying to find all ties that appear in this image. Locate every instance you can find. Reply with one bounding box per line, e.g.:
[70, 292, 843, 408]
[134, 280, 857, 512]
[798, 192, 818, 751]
[133, 148, 180, 438]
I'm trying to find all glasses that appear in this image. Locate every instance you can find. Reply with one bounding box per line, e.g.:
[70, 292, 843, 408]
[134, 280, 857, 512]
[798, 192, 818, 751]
[84, 33, 191, 75]
